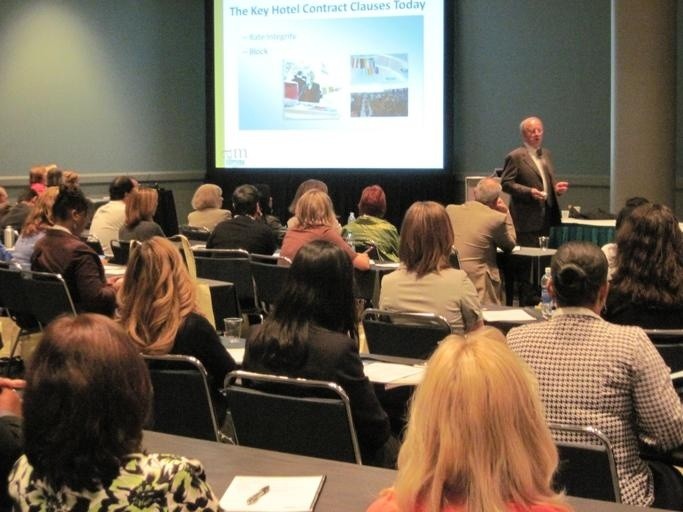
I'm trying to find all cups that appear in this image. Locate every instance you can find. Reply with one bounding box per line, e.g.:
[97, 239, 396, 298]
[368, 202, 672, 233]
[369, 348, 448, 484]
[561, 210, 569, 221]
[224, 318, 242, 344]
[538, 237, 549, 252]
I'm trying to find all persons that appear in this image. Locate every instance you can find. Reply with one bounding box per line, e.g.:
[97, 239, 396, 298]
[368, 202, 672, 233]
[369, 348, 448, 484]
[111, 237, 241, 428]
[238, 239, 401, 467]
[0, 375, 29, 512]
[504, 241, 683, 510]
[8, 311, 225, 511]
[0, 158, 682, 392]
[355, 333, 575, 512]
[504, 117, 570, 286]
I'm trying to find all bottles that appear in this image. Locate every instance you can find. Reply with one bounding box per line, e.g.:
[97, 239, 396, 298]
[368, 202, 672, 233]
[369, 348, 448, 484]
[346, 231, 355, 252]
[2, 226, 15, 248]
[347, 212, 355, 225]
[541, 268, 553, 309]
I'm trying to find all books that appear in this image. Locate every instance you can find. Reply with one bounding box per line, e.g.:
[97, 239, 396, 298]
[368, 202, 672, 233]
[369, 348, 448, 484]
[218, 473, 329, 511]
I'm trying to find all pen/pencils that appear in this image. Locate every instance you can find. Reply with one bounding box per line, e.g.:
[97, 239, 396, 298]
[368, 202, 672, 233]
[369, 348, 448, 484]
[364, 246, 374, 254]
[246, 485, 270, 504]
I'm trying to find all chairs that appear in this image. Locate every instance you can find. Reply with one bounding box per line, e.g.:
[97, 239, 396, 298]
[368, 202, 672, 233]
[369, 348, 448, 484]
[0, 226, 683, 503]
